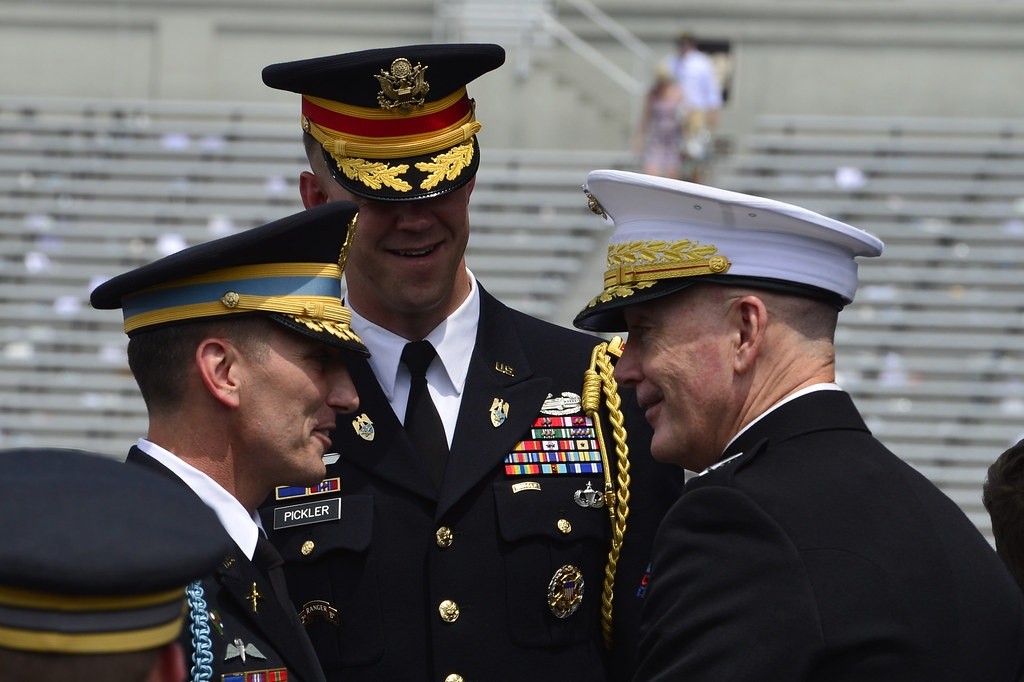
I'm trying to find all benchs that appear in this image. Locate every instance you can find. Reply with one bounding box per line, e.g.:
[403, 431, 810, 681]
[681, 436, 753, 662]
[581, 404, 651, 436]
[0, 95, 1024, 553]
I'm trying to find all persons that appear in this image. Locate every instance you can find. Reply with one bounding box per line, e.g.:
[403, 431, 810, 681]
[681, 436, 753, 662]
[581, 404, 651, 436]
[92, 32, 1023, 681]
[0, 447, 228, 682]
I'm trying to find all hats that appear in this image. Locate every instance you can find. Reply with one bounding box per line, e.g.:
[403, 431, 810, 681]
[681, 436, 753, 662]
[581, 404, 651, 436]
[0, 449, 227, 654]
[573, 169, 883, 335]
[89, 202, 370, 357]
[261, 43, 506, 200]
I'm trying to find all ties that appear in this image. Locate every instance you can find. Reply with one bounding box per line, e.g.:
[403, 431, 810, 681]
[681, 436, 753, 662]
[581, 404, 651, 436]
[400, 340, 450, 493]
[253, 529, 327, 630]
